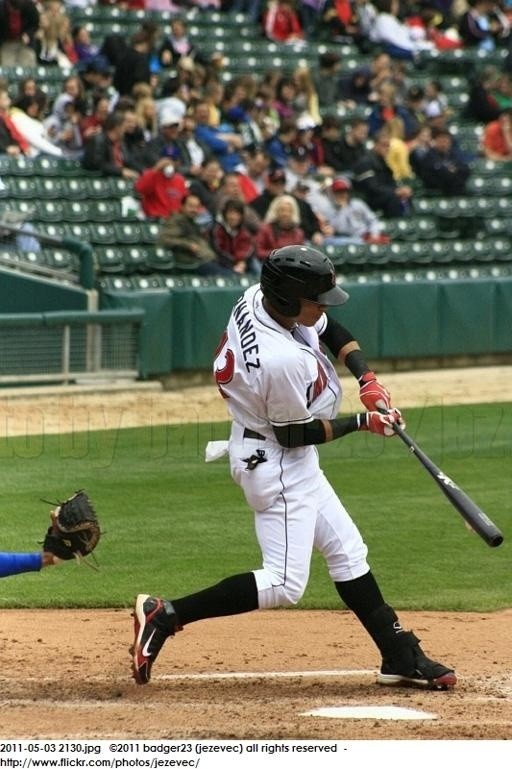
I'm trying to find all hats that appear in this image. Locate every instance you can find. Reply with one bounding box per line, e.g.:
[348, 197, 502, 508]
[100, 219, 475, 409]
[333, 180, 349, 189]
[158, 114, 177, 126]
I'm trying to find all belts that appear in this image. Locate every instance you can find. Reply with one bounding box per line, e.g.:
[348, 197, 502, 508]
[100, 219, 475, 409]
[242, 424, 267, 441]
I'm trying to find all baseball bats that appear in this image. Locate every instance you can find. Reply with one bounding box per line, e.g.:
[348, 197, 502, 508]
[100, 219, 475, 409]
[383, 410, 504, 547]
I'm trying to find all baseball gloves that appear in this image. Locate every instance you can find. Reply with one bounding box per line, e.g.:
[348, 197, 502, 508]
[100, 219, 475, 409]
[37, 489, 102, 573]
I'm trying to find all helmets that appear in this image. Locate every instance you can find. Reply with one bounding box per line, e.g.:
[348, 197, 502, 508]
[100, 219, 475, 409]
[259, 244, 351, 319]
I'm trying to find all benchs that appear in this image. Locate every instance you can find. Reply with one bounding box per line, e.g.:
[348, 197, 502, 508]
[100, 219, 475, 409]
[0, 7, 512, 295]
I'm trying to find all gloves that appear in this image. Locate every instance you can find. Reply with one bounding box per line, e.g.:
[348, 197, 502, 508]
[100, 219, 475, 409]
[357, 370, 392, 413]
[355, 406, 407, 438]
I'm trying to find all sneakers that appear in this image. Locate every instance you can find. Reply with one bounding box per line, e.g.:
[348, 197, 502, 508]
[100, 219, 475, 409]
[127, 593, 186, 685]
[376, 630, 457, 689]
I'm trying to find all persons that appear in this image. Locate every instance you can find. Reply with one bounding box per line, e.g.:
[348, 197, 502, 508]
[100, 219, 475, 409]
[1, 0, 511, 275]
[128, 244, 458, 692]
[1, 487, 101, 580]
